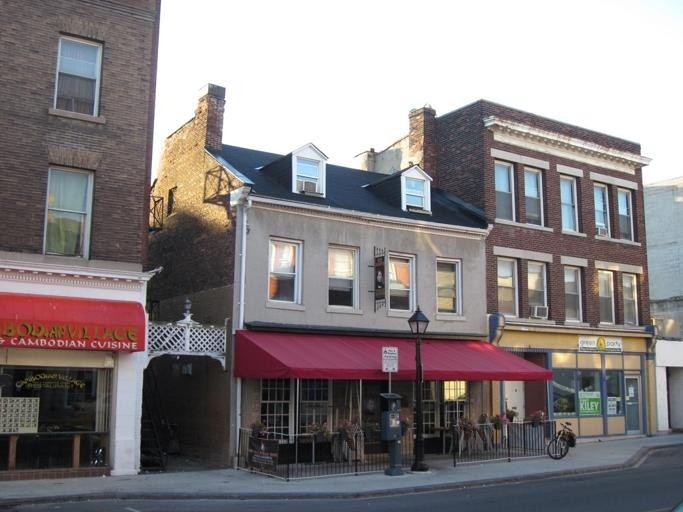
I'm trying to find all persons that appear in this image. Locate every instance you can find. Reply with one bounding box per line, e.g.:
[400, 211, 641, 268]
[365, 398, 375, 416]
[376, 270, 384, 288]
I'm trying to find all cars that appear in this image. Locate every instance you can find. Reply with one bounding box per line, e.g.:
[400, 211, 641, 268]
[70, 392, 111, 416]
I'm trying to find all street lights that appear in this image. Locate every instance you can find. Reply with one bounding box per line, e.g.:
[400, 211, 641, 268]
[407, 306, 431, 470]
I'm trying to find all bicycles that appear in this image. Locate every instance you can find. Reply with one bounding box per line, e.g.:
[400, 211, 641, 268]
[546, 422, 577, 460]
